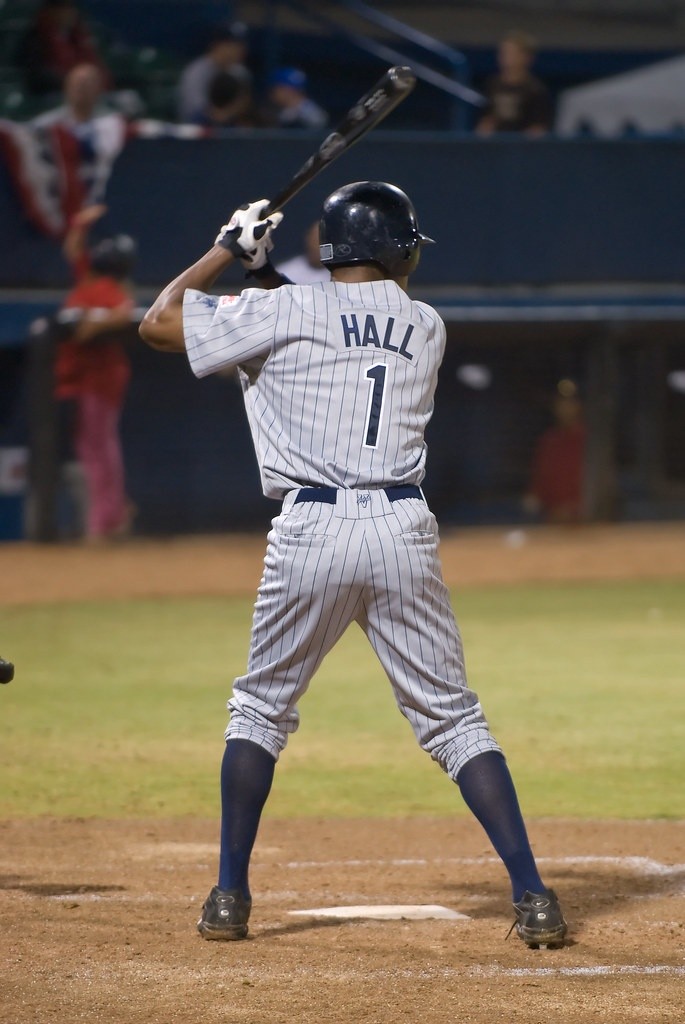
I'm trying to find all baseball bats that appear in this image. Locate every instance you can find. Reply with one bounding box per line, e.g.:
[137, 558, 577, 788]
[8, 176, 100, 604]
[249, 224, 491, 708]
[252, 62, 421, 226]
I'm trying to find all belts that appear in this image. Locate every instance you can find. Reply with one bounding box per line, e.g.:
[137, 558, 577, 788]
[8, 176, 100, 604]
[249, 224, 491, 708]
[283, 486, 427, 502]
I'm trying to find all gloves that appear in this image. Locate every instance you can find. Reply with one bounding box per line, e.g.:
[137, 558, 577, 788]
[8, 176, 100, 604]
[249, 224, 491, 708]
[234, 236, 283, 271]
[216, 199, 284, 253]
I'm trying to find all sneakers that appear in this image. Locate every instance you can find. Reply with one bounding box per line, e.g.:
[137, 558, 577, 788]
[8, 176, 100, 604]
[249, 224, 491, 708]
[505, 886, 568, 949]
[197, 884, 253, 941]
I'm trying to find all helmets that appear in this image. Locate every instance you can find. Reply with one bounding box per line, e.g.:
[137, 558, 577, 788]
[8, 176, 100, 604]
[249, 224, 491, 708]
[315, 178, 435, 276]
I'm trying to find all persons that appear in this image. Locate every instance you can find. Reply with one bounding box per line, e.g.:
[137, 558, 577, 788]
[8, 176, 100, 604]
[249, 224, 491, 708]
[0, 0, 621, 542]
[140, 181, 569, 948]
